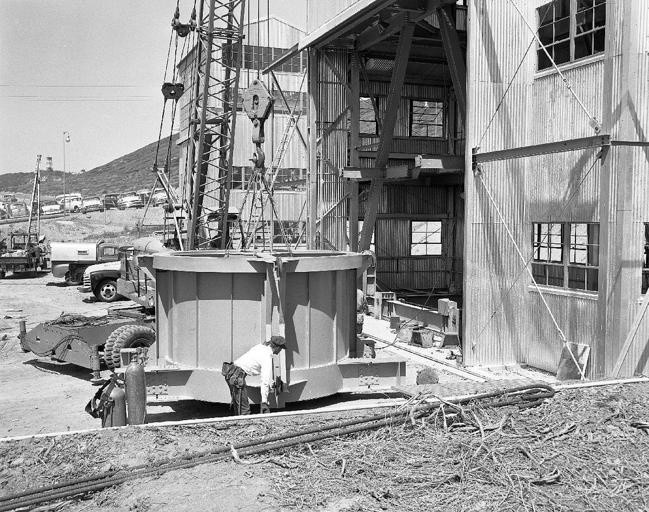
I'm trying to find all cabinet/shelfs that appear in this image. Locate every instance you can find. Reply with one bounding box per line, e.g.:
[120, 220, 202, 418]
[362, 265, 376, 308]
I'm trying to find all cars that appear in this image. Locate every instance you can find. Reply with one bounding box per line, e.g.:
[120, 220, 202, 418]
[3, 189, 169, 217]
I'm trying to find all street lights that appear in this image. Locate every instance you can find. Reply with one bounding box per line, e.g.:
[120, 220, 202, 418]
[63, 131, 72, 214]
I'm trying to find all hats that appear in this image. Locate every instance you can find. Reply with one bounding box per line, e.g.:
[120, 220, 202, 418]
[270, 335, 288, 350]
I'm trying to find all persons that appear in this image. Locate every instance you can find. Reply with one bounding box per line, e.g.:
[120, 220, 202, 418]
[220, 335, 288, 414]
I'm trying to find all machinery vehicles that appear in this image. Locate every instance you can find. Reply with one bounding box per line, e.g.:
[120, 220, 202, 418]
[1, 153, 50, 276]
[19, 238, 173, 371]
